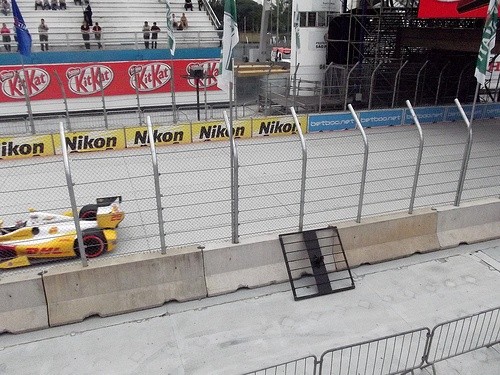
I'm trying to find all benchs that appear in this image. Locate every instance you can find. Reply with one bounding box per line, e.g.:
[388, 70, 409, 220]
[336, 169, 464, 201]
[0, 0, 221, 52]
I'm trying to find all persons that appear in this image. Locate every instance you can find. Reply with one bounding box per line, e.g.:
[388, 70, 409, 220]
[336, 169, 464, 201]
[142, 20, 151, 49]
[184, 0, 193, 11]
[198, 0, 204, 11]
[151, 20, 161, 49]
[34, 0, 67, 11]
[80, 20, 91, 49]
[37, 19, 49, 51]
[171, 13, 179, 27]
[181, 12, 188, 30]
[83, 0, 93, 26]
[0, 0, 11, 16]
[92, 22, 103, 49]
[0, 23, 11, 52]
[74, 0, 81, 5]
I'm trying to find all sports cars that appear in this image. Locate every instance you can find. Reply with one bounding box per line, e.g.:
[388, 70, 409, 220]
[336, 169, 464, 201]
[0, 195, 124, 270]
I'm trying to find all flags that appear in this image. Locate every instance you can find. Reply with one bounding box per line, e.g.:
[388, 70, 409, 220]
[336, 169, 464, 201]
[473, 0, 498, 87]
[10, 0, 32, 57]
[217, 0, 240, 94]
